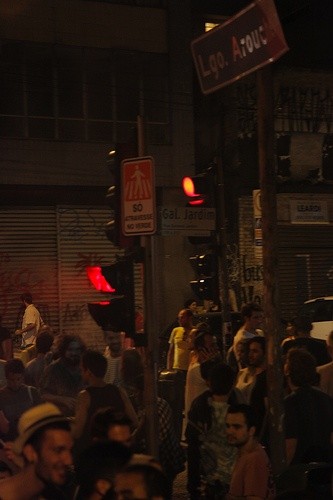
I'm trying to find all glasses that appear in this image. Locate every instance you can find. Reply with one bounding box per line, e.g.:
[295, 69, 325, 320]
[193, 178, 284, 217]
[176, 317, 187, 321]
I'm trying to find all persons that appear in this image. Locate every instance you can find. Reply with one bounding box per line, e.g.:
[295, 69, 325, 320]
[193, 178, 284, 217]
[0, 292, 333, 500]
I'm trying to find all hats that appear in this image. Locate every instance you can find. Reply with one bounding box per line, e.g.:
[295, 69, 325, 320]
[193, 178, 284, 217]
[11, 403, 79, 454]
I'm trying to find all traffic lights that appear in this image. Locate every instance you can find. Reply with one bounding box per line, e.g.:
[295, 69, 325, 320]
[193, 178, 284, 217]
[104, 143, 139, 245]
[82, 256, 136, 333]
[176, 163, 220, 208]
[185, 230, 220, 300]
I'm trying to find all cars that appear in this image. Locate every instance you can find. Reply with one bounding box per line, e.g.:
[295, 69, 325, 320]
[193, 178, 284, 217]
[290, 296, 332, 343]
